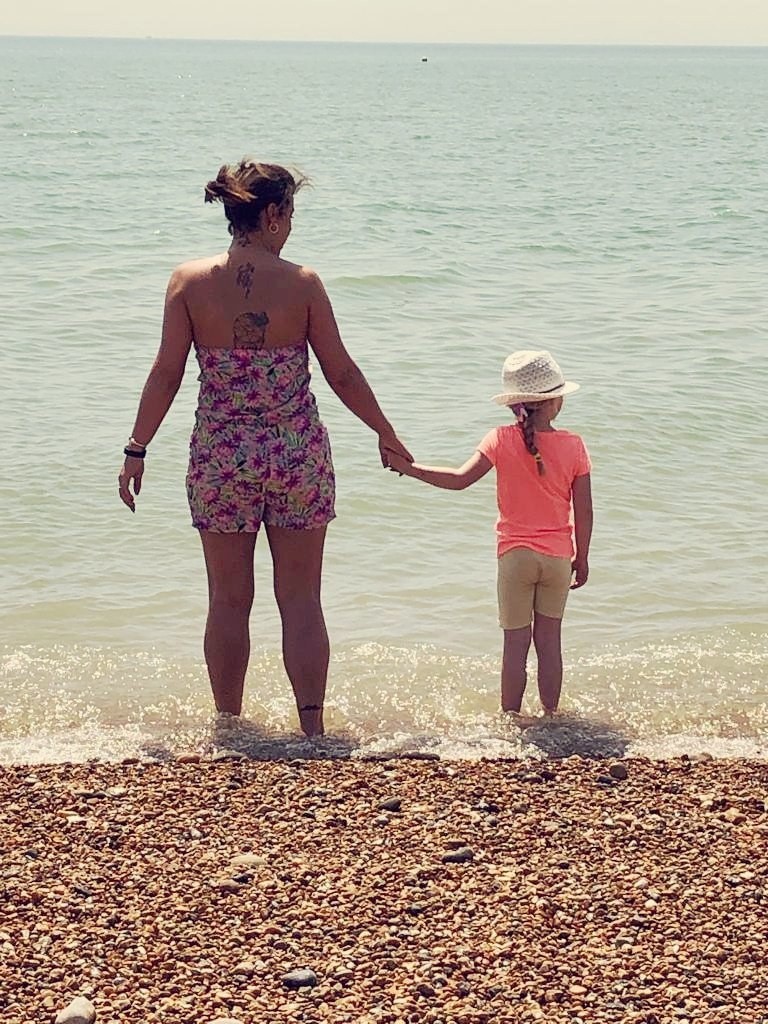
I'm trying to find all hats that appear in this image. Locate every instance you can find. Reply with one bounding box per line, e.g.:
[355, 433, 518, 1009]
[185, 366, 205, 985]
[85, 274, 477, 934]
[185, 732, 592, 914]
[490, 350, 580, 408]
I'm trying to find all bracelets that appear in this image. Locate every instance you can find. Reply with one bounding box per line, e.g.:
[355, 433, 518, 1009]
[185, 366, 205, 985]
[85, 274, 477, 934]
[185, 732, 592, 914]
[124, 446, 147, 458]
[129, 436, 147, 448]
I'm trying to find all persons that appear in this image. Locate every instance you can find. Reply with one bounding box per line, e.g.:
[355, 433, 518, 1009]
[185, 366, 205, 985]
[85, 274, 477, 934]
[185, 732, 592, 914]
[116, 152, 416, 738]
[382, 348, 593, 716]
